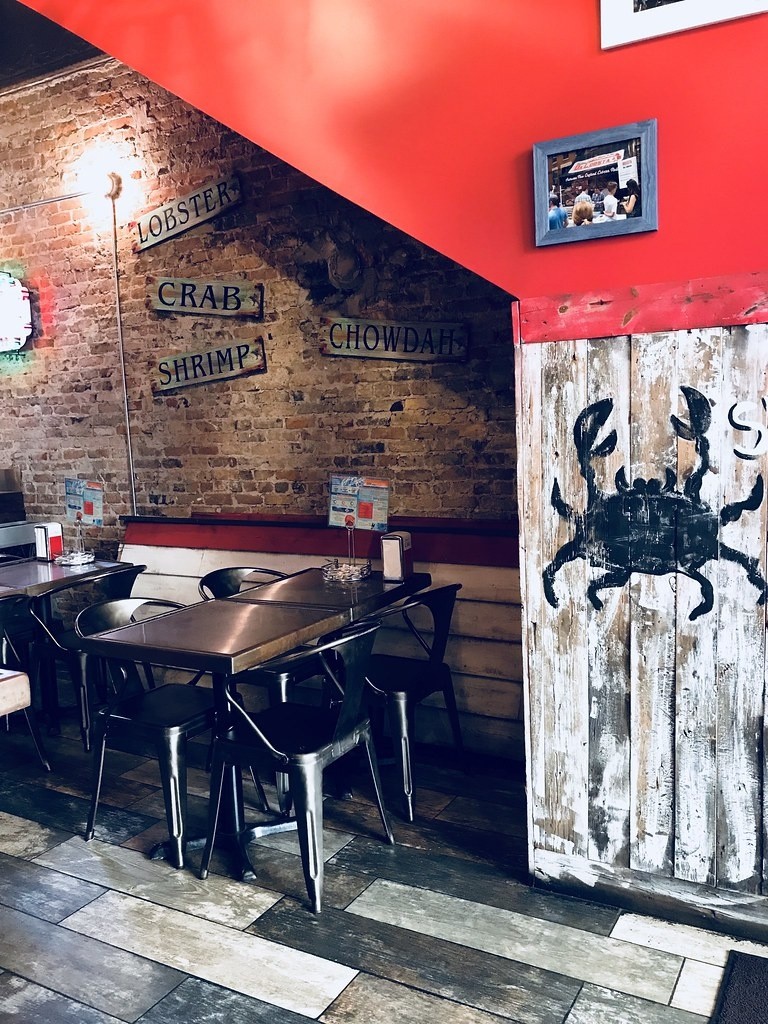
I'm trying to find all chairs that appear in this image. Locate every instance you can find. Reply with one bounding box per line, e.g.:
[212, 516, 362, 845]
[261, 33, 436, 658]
[316, 582, 466, 823]
[197, 566, 348, 819]
[28, 565, 149, 752]
[0, 594, 52, 772]
[73, 596, 270, 870]
[199, 619, 397, 914]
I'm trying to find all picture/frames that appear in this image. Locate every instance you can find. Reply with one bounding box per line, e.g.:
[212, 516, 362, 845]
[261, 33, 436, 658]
[599, 0, 768, 51]
[532, 117, 658, 247]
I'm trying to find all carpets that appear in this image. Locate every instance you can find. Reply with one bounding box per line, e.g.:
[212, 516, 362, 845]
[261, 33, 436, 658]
[707, 949, 768, 1024]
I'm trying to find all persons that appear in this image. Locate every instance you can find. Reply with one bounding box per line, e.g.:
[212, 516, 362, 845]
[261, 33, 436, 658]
[548, 175, 642, 229]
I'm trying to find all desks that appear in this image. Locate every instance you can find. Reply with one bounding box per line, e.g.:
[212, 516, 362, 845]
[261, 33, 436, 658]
[226, 567, 435, 623]
[0, 586, 26, 605]
[0, 553, 134, 737]
[81, 597, 349, 883]
